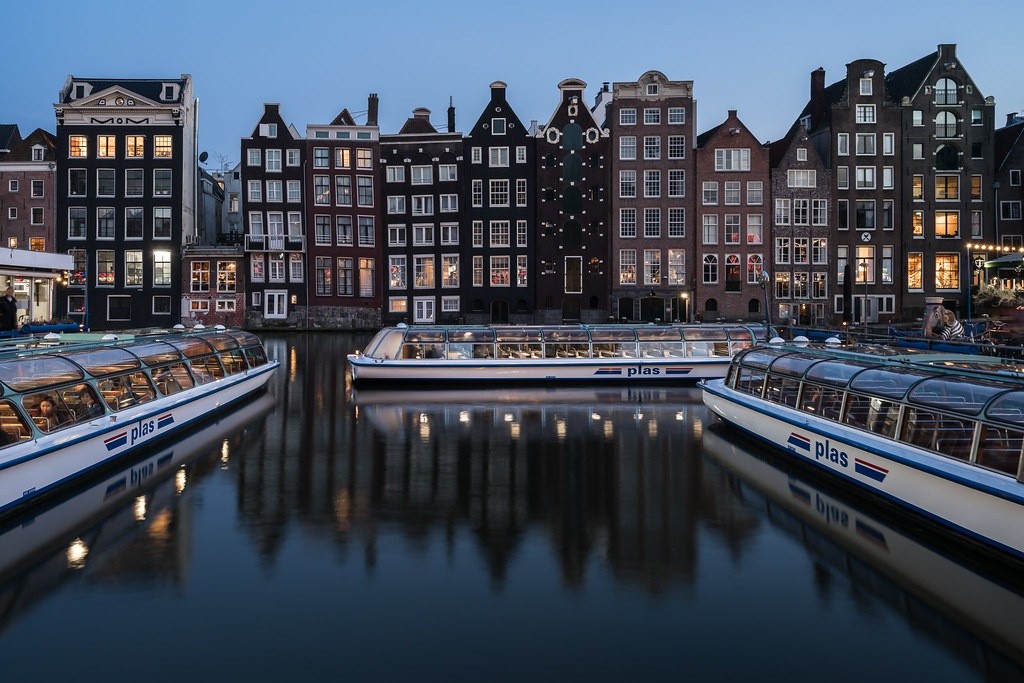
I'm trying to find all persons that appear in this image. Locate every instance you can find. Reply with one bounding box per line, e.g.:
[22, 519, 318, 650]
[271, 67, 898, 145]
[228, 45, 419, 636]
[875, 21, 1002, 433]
[404, 332, 753, 364]
[0, 358, 170, 451]
[0, 287, 18, 349]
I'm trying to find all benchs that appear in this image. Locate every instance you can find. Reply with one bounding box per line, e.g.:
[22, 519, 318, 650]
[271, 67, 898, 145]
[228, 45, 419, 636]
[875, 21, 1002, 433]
[0, 355, 256, 442]
[736, 377, 1024, 480]
[472, 343, 745, 359]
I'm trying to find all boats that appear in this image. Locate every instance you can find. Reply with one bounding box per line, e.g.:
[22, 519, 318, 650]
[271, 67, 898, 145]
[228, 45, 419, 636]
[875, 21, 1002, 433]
[696, 340, 1024, 559]
[346, 322, 781, 382]
[1, 324, 281, 510]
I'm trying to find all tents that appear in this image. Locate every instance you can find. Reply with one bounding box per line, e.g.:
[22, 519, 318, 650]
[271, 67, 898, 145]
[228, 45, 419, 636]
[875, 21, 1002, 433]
[984, 252, 1024, 289]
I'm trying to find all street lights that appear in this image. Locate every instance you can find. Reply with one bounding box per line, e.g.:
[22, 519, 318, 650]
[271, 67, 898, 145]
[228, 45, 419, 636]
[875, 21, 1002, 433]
[974, 253, 985, 293]
